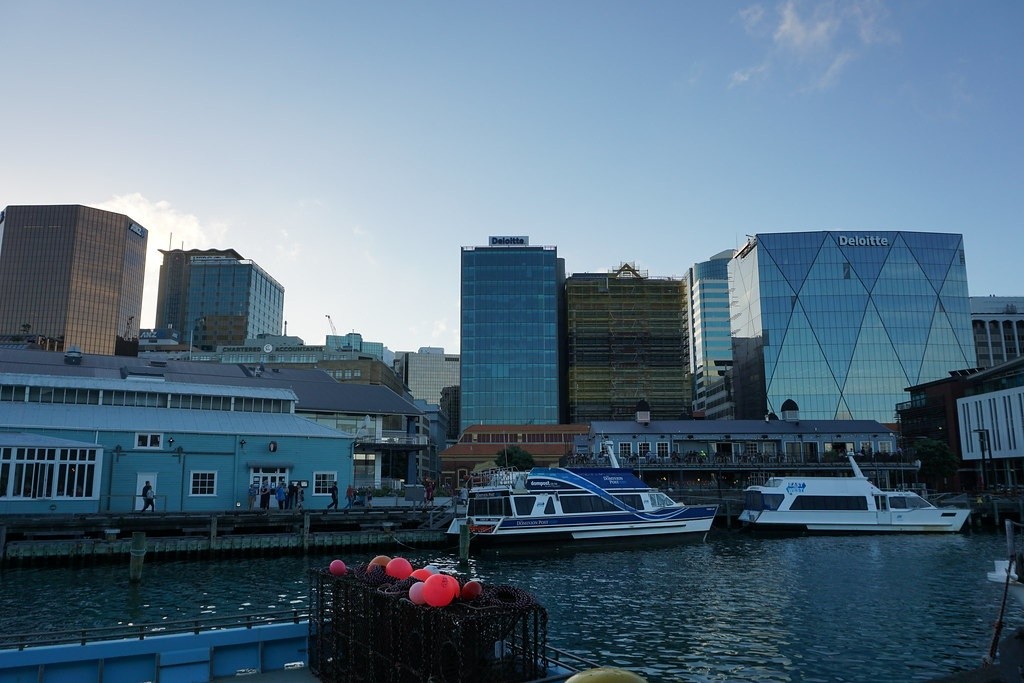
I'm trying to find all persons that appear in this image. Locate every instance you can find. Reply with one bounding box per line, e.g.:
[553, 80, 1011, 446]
[327, 481, 338, 508]
[629, 452, 638, 464]
[260, 480, 270, 510]
[671, 449, 706, 464]
[249, 484, 256, 509]
[575, 450, 606, 464]
[275, 481, 304, 510]
[141, 481, 155, 512]
[419, 480, 435, 506]
[461, 485, 468, 507]
[367, 485, 373, 508]
[344, 485, 353, 508]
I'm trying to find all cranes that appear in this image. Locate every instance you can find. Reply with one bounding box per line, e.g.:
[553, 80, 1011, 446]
[326, 315, 343, 352]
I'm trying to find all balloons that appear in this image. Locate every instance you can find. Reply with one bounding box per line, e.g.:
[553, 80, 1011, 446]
[366, 555, 482, 608]
[330, 560, 346, 575]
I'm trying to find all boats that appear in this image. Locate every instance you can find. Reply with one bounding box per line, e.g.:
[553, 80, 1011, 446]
[446, 441, 719, 550]
[738, 452, 971, 535]
